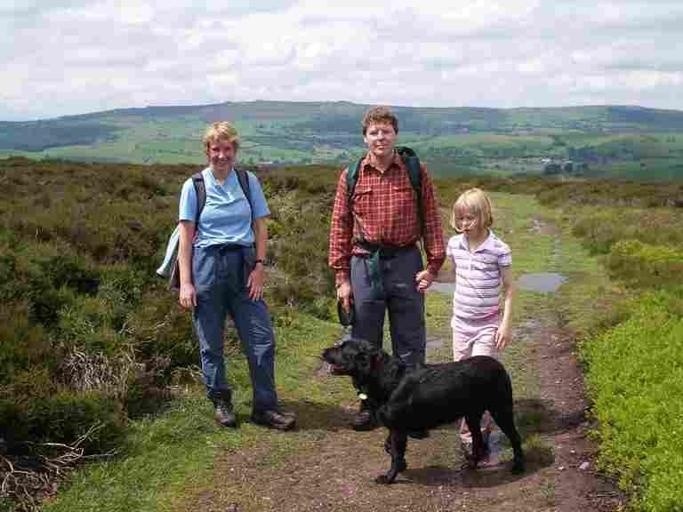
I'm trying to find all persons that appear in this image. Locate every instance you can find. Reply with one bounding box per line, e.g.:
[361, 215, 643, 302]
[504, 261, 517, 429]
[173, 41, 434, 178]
[178, 120, 296, 432]
[416, 187, 513, 455]
[328, 106, 446, 439]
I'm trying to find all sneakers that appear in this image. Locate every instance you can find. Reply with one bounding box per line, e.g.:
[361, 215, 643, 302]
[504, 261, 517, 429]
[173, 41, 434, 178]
[462, 428, 492, 461]
[408, 429, 430, 439]
[252, 408, 296, 429]
[215, 406, 236, 426]
[353, 410, 375, 430]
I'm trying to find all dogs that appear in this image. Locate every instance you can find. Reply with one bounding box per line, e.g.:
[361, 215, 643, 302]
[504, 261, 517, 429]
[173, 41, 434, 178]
[318, 336, 526, 485]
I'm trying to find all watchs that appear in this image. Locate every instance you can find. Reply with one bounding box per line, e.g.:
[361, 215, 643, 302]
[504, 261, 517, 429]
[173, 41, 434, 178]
[254, 258, 268, 266]
